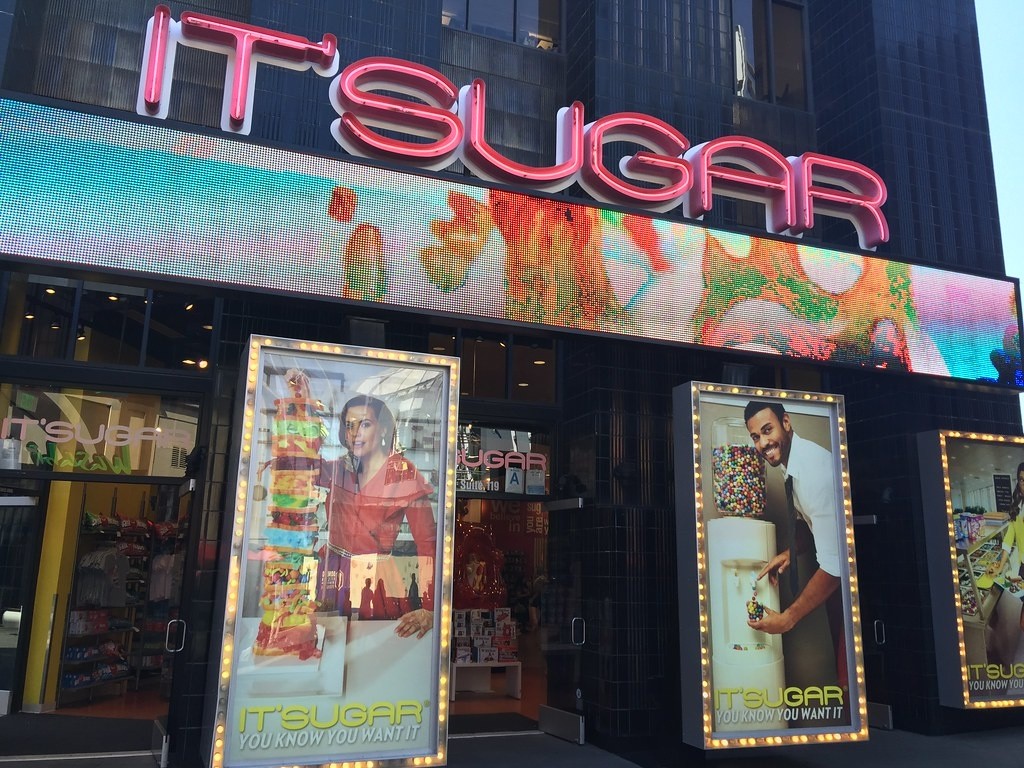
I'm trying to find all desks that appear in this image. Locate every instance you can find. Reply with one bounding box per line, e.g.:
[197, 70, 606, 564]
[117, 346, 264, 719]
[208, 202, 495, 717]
[449, 661, 522, 701]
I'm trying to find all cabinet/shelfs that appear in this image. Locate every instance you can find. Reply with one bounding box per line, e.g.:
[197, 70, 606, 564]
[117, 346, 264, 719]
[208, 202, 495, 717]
[55, 484, 156, 709]
[133, 531, 184, 694]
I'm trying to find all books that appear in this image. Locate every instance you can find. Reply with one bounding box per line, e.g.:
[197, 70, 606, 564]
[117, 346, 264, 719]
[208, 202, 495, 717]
[452, 607, 519, 663]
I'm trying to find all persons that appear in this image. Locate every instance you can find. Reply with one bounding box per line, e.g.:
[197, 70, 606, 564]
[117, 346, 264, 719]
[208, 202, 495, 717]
[744, 401, 847, 678]
[524, 579, 541, 632]
[985, 462, 1024, 631]
[282, 368, 437, 641]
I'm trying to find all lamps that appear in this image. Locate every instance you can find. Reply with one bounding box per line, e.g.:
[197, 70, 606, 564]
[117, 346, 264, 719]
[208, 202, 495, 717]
[51, 311, 60, 329]
[25, 307, 35, 319]
[77, 322, 85, 341]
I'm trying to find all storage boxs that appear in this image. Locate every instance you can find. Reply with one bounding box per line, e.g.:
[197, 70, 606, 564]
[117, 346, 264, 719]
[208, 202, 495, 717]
[452, 607, 517, 663]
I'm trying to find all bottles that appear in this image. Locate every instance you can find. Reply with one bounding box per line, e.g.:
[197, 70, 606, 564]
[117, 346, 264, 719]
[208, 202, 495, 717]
[710, 417, 768, 519]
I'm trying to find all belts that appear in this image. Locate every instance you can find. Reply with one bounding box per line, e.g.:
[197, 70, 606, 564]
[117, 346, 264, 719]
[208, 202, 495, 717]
[327, 542, 393, 562]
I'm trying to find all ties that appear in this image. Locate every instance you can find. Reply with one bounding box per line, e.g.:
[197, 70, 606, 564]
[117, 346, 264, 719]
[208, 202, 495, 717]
[784, 475, 799, 596]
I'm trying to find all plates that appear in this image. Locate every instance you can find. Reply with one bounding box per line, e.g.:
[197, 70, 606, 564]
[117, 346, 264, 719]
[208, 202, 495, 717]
[994, 575, 1024, 598]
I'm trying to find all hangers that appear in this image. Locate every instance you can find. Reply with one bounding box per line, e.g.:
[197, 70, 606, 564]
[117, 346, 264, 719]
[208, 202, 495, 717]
[91, 538, 117, 557]
